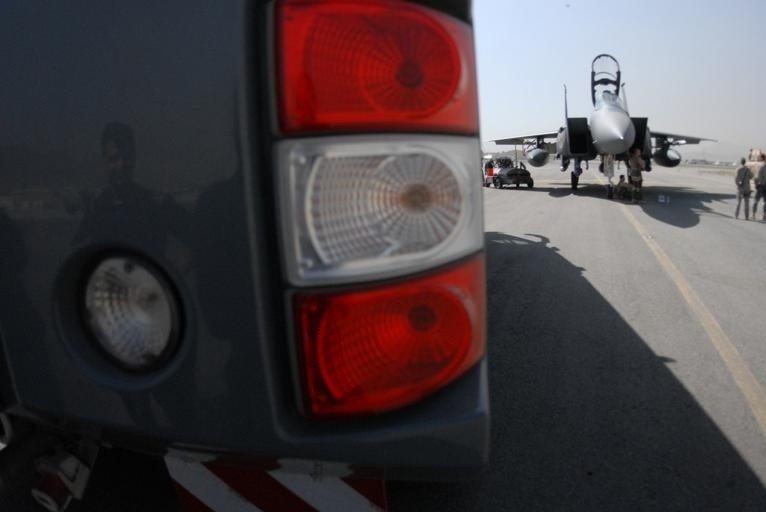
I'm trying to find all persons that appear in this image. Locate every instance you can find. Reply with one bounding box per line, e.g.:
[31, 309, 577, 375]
[613, 174, 639, 200]
[750, 154, 765, 222]
[627, 148, 649, 205]
[733, 157, 754, 221]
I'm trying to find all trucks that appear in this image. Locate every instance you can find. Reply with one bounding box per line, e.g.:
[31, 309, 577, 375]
[2, 1, 502, 511]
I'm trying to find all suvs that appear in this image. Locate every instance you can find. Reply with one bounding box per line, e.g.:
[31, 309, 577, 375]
[481, 155, 534, 189]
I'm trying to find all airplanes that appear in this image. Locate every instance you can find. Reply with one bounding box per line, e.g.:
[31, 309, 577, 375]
[483, 52, 718, 202]
